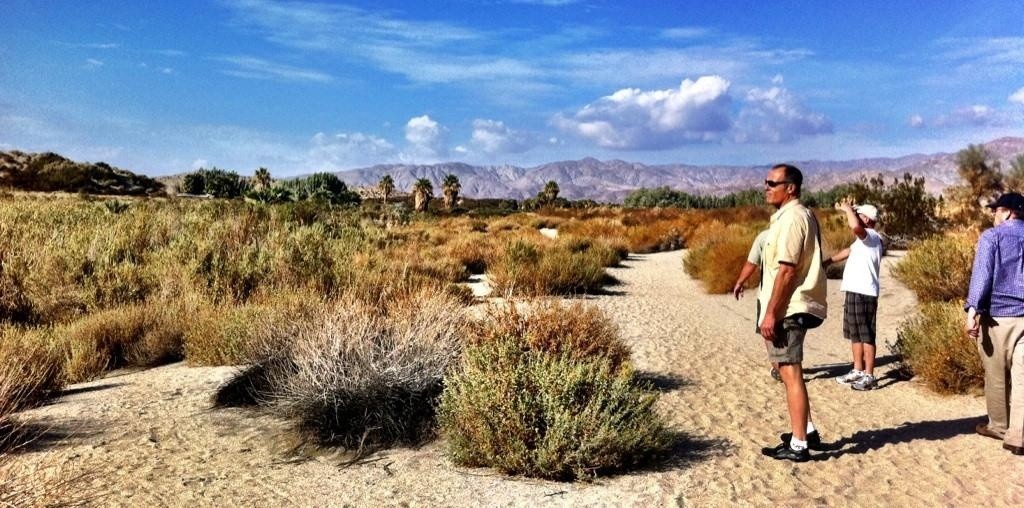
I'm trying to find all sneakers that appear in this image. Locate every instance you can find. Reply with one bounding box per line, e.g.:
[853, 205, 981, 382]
[851, 375, 879, 391]
[835, 369, 865, 384]
[771, 368, 781, 381]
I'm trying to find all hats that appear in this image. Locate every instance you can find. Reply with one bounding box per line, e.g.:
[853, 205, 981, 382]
[856, 204, 878, 222]
[985, 193, 1024, 211]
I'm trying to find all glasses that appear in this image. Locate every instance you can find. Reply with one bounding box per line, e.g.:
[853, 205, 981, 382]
[765, 179, 792, 187]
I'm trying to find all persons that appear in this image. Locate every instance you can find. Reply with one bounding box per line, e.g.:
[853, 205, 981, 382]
[734, 229, 787, 381]
[760, 164, 827, 461]
[820, 198, 885, 390]
[963, 190, 1024, 455]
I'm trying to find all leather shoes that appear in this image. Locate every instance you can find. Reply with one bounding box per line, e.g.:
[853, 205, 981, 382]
[976, 422, 1005, 440]
[780, 429, 820, 448]
[762, 442, 809, 462]
[1003, 443, 1024, 455]
